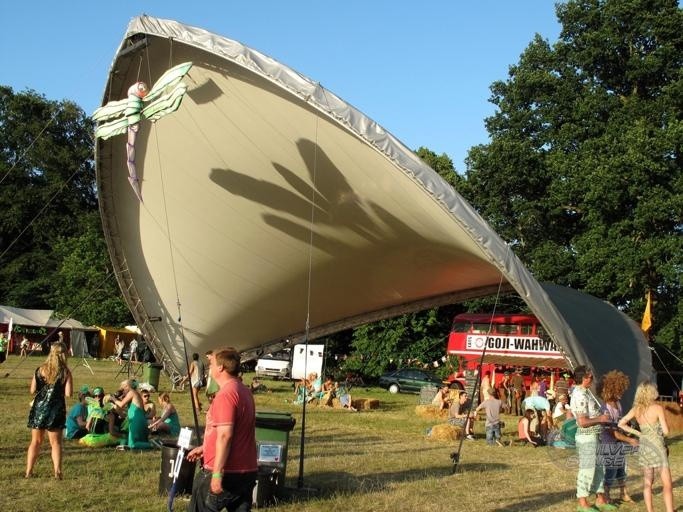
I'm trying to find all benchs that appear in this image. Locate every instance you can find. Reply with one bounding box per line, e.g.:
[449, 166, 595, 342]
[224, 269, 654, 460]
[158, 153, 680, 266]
[641, 292, 651, 331]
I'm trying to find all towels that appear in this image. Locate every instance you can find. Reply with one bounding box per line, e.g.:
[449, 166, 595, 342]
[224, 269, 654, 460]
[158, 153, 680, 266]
[141, 362, 163, 392]
[255, 412, 296, 488]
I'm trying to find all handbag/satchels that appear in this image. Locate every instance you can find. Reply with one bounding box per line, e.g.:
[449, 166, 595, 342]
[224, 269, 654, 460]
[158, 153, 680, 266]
[630, 428, 638, 435]
[211, 473, 223, 478]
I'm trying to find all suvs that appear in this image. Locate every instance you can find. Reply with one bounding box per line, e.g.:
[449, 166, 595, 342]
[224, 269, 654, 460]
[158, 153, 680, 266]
[158, 443, 197, 497]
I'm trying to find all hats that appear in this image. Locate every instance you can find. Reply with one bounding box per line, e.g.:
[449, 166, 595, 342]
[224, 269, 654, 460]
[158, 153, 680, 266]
[377, 367, 449, 395]
[254, 348, 291, 378]
[330, 352, 347, 361]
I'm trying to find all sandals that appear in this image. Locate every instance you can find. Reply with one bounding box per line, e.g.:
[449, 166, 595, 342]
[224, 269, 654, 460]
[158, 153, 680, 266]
[446, 312, 570, 372]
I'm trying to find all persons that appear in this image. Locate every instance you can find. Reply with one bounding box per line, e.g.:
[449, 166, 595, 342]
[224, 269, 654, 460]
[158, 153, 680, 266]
[570, 366, 618, 512]
[187, 346, 259, 512]
[0, 331, 220, 450]
[432, 370, 573, 448]
[238, 367, 358, 412]
[24, 341, 73, 480]
[595, 369, 639, 505]
[618, 380, 674, 512]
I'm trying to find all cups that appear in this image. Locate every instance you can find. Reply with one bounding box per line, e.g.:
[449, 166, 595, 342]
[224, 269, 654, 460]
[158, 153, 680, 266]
[587, 375, 593, 380]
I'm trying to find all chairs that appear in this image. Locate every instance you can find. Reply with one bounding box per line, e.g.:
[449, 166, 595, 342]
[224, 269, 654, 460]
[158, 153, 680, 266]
[466, 434, 475, 441]
[577, 503, 598, 512]
[594, 503, 617, 512]
